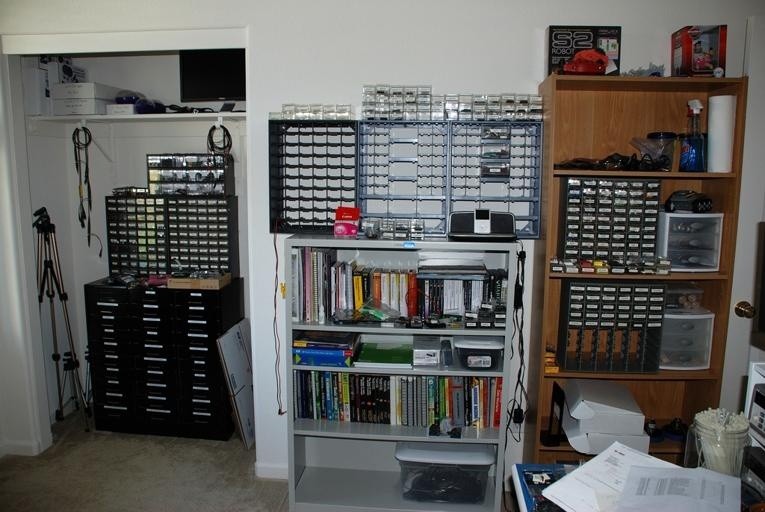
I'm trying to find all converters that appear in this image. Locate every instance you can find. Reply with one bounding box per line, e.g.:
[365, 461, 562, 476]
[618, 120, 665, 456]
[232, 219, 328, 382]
[512, 408, 523, 423]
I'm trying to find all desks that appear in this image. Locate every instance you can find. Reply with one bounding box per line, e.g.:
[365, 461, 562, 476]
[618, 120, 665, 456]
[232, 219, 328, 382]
[511, 460, 750, 512]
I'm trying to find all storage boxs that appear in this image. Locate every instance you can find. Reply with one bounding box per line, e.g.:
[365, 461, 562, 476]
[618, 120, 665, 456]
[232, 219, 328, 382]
[658, 306, 715, 372]
[562, 406, 651, 455]
[654, 209, 725, 272]
[559, 381, 644, 435]
[549, 25, 621, 75]
[671, 25, 727, 79]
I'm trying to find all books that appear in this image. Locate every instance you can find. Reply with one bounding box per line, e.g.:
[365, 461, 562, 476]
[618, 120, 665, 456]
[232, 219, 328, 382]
[511, 463, 582, 512]
[293, 330, 502, 429]
[291, 246, 507, 329]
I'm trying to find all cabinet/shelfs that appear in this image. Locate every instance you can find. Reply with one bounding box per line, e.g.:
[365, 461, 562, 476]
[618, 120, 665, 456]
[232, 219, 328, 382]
[84, 276, 243, 442]
[524, 71, 745, 462]
[269, 99, 542, 512]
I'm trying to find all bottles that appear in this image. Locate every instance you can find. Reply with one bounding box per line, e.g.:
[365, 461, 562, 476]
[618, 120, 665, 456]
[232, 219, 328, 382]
[682, 413, 753, 483]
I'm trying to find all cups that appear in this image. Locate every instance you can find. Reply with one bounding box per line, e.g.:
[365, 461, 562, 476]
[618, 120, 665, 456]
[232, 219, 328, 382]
[646, 131, 676, 171]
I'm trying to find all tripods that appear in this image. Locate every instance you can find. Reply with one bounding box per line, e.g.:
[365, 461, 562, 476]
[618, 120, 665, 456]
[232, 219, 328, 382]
[30, 206, 91, 432]
